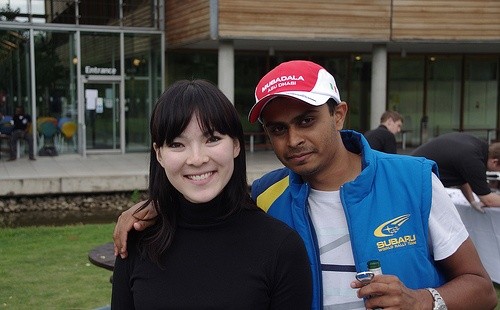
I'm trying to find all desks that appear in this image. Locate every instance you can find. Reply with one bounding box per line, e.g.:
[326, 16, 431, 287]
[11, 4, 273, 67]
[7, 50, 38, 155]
[446, 189, 500, 284]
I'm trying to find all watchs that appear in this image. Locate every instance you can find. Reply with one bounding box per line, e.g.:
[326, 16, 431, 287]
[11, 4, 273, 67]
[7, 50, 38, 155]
[425, 287, 448, 310]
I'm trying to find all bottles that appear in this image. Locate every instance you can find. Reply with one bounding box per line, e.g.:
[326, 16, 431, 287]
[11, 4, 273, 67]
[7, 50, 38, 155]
[367, 260, 383, 310]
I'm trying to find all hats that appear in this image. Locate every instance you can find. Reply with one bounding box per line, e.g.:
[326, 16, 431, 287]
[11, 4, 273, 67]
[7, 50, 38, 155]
[248, 60, 341, 128]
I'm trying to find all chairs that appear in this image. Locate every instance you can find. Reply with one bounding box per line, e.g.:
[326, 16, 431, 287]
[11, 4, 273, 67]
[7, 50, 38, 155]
[0, 112, 77, 160]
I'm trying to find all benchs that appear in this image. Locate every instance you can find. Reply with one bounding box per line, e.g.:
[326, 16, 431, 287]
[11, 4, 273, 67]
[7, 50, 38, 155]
[399, 130, 412, 149]
[452, 128, 500, 146]
[242, 131, 269, 153]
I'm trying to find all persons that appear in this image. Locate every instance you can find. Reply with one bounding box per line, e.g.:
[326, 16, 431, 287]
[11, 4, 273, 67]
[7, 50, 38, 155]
[361, 109, 403, 154]
[110, 79, 315, 310]
[7, 105, 38, 162]
[111, 58, 499, 310]
[405, 130, 500, 214]
